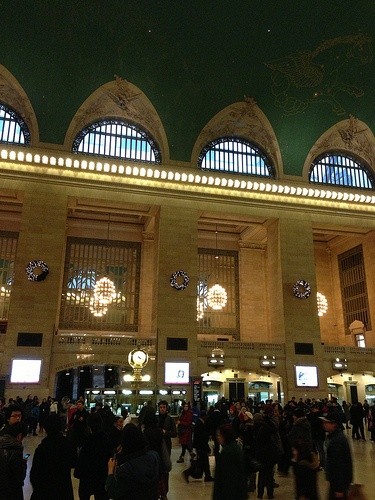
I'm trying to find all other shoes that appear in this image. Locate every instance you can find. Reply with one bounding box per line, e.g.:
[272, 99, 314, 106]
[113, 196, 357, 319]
[190, 453, 196, 461]
[183, 471, 189, 483]
[177, 458, 184, 462]
[204, 477, 214, 481]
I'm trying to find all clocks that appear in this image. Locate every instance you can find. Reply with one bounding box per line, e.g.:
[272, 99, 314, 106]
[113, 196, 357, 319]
[127, 349, 148, 380]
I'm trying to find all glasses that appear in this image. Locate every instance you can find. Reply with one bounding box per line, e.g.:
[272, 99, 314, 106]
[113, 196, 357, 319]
[10, 414, 22, 418]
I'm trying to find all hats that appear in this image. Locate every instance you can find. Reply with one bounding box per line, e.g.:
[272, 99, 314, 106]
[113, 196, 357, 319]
[317, 412, 339, 423]
[293, 408, 305, 417]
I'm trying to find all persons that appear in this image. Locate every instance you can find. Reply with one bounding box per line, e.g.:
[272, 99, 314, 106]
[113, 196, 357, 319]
[0, 395, 375, 500]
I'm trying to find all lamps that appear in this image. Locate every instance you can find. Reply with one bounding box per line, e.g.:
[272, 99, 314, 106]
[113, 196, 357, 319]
[317, 292, 328, 317]
[196, 223, 228, 321]
[90, 215, 117, 317]
